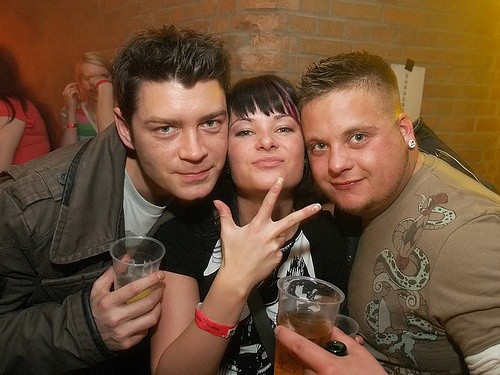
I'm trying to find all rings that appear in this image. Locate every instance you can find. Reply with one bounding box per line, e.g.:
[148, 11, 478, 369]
[67, 89, 70, 92]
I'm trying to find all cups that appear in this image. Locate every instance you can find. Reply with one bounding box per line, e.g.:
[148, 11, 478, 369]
[110, 235, 167, 318]
[273, 275, 345, 375]
[333, 314, 359, 340]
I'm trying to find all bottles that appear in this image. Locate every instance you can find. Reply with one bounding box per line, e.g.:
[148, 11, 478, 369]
[324, 340, 348, 357]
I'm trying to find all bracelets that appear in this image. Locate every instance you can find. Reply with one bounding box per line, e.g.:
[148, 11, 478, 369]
[66, 123, 78, 128]
[96, 79, 111, 89]
[195, 304, 239, 339]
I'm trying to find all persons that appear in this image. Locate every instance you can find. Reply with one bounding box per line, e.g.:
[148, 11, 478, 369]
[275, 51, 500, 375]
[90, 76, 115, 134]
[0, 25, 231, 375]
[59, 52, 111, 147]
[150, 73, 350, 375]
[0, 44, 50, 172]
